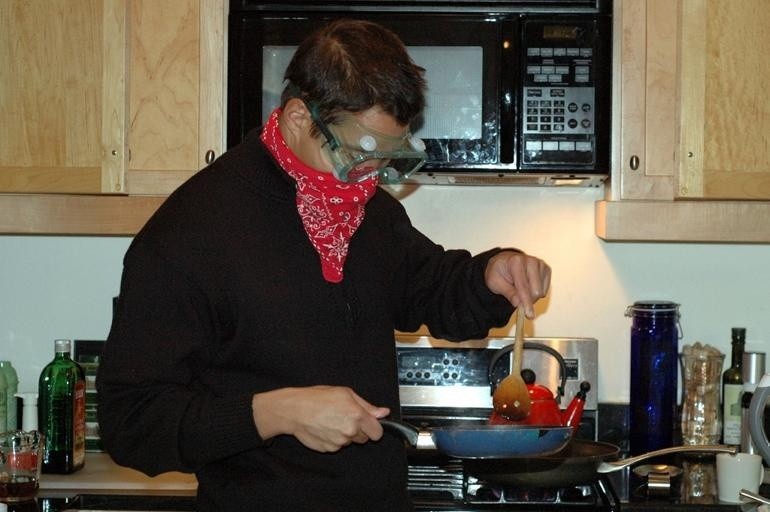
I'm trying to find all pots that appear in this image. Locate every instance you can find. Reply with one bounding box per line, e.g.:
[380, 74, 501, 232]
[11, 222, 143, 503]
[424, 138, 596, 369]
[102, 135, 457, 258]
[467, 440, 738, 492]
[378, 419, 576, 460]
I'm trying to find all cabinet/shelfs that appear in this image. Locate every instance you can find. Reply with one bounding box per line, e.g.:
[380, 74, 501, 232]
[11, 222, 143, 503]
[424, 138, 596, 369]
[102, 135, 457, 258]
[595, 1, 770, 249]
[1, 1, 230, 239]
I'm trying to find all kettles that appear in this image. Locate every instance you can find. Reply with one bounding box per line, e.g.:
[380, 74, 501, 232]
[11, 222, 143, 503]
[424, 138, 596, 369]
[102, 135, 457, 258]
[486, 338, 595, 427]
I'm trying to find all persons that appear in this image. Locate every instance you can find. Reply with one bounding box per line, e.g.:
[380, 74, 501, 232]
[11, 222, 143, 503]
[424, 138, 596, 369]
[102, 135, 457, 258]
[97, 21, 554, 510]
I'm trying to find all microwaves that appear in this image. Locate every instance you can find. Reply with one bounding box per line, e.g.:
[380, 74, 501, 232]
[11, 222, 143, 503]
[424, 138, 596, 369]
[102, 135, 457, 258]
[227, 1, 610, 189]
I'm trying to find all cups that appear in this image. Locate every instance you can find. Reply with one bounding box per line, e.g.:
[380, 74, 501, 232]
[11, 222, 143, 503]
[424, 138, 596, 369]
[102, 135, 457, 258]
[716, 453, 764, 506]
[0, 430, 46, 504]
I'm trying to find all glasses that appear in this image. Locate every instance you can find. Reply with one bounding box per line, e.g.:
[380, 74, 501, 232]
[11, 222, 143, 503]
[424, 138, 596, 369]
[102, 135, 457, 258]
[302, 94, 429, 187]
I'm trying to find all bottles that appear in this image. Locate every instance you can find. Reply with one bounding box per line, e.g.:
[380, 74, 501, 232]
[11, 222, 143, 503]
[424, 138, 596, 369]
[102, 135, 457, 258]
[724, 327, 747, 449]
[741, 353, 765, 452]
[679, 344, 724, 454]
[37, 339, 86, 474]
[625, 302, 683, 452]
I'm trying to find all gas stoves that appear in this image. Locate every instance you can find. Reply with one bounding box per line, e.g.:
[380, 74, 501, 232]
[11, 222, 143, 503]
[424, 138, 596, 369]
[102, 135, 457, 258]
[400, 447, 624, 512]
